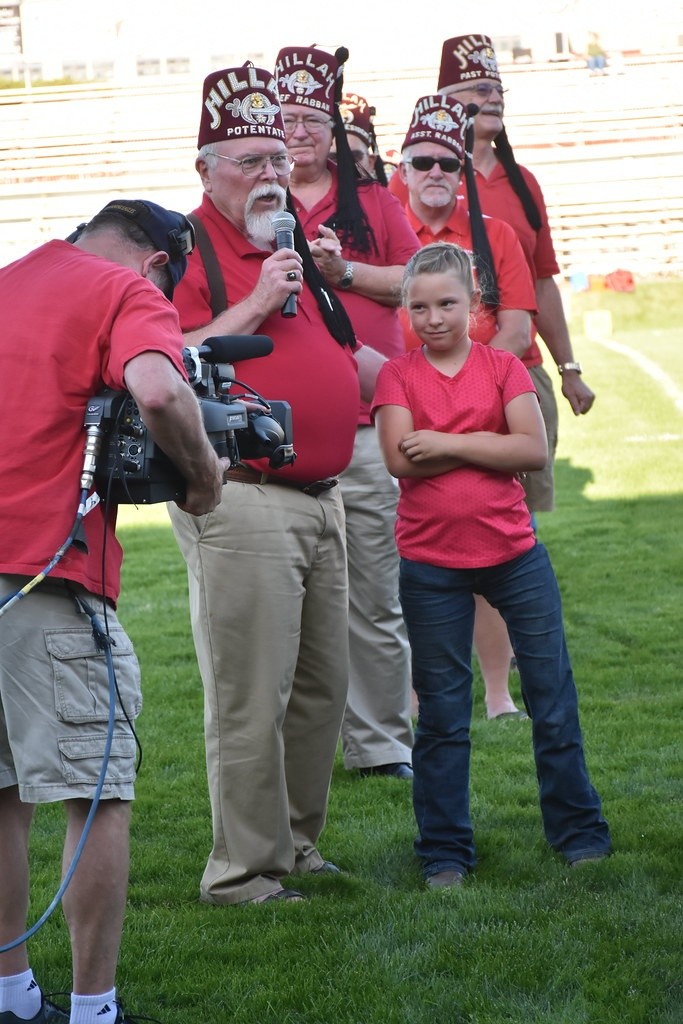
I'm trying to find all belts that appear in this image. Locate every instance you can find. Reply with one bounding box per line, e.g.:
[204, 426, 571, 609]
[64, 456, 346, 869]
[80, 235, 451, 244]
[223, 469, 339, 499]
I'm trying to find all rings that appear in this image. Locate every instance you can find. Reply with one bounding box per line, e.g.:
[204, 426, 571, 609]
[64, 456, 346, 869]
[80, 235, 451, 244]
[286, 269, 297, 283]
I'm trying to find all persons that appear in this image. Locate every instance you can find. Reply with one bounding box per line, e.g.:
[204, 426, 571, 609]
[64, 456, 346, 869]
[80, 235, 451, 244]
[0, 200, 231, 1023]
[164, 33, 614, 909]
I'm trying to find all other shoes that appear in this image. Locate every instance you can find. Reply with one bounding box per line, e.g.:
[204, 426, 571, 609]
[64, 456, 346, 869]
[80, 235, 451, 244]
[571, 855, 609, 867]
[261, 890, 310, 907]
[315, 862, 352, 879]
[0, 990, 71, 1024]
[425, 872, 465, 896]
[496, 710, 530, 724]
[112, 998, 162, 1024]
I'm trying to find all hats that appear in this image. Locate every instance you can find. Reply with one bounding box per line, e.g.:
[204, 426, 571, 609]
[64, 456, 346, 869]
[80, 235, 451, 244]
[99, 199, 197, 301]
[334, 92, 389, 187]
[198, 60, 358, 350]
[274, 46, 380, 256]
[436, 34, 541, 231]
[400, 94, 498, 307]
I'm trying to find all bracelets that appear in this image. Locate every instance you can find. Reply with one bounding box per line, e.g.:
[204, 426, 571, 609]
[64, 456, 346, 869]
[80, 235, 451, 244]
[558, 361, 582, 375]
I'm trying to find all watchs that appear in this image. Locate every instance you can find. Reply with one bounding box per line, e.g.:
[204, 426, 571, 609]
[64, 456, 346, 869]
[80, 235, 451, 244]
[336, 261, 353, 289]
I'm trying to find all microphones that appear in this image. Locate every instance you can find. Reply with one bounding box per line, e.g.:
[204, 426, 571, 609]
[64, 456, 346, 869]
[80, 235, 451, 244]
[182, 334, 274, 364]
[271, 212, 296, 317]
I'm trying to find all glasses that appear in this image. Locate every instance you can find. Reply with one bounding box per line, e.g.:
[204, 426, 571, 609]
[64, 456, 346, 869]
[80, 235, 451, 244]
[449, 82, 509, 96]
[279, 115, 331, 135]
[206, 151, 299, 178]
[404, 156, 460, 172]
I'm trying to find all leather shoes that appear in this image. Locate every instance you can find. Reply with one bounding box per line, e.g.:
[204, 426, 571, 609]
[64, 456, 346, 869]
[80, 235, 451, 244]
[360, 763, 415, 780]
